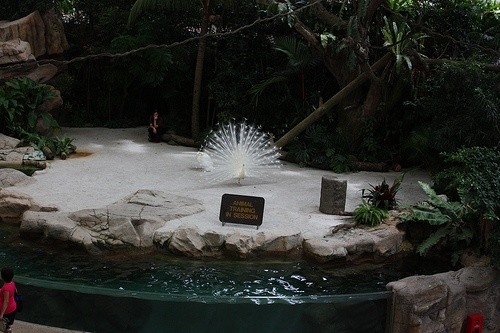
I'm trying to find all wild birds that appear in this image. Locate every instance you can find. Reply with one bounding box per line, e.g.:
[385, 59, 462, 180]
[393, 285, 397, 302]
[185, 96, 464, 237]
[195, 117, 284, 186]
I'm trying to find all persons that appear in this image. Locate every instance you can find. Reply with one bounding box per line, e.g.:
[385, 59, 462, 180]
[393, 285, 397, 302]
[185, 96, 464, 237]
[147, 111, 167, 142]
[0, 267, 20, 333]
